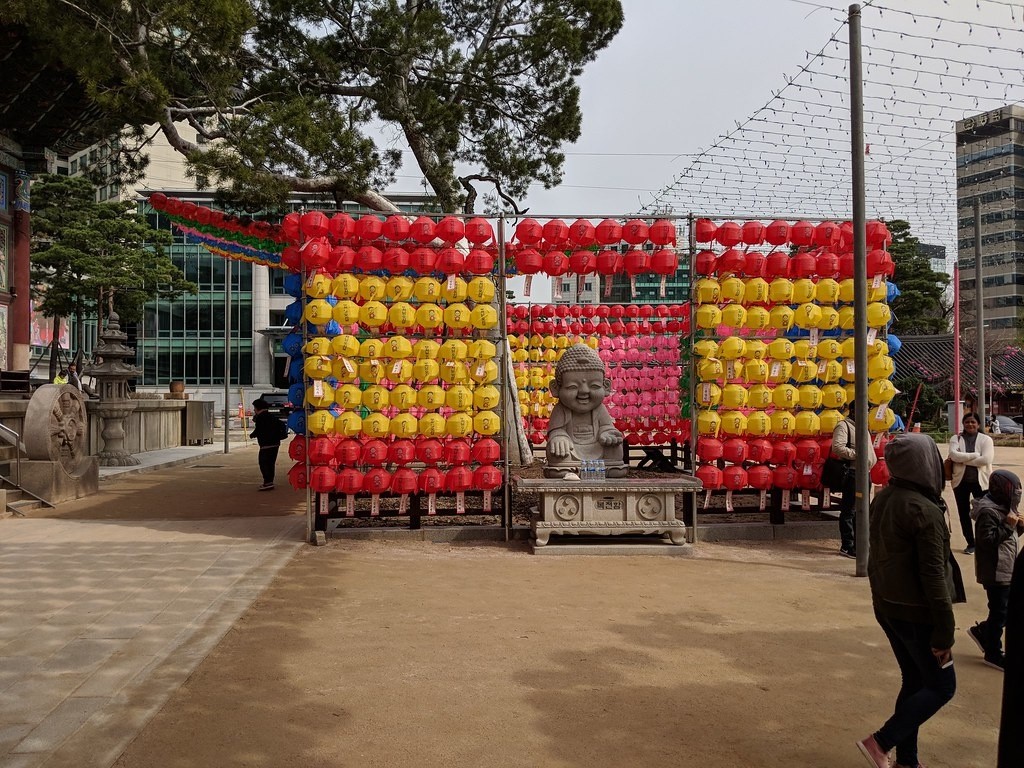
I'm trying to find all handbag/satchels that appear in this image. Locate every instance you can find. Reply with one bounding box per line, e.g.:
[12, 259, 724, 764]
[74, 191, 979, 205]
[819, 420, 853, 493]
[943, 435, 960, 481]
[277, 419, 288, 440]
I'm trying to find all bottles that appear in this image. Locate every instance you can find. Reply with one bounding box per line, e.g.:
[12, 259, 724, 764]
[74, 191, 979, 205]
[581, 460, 605, 481]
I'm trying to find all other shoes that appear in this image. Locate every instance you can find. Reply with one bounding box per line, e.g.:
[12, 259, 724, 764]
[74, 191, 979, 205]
[892, 760, 928, 768]
[967, 620, 985, 653]
[847, 551, 856, 559]
[258, 482, 274, 489]
[983, 650, 1005, 671]
[89, 394, 99, 399]
[963, 546, 974, 555]
[839, 546, 848, 555]
[856, 735, 891, 768]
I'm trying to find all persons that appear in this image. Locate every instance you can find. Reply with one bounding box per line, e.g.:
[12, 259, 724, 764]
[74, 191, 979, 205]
[66, 362, 100, 399]
[545, 343, 624, 466]
[53, 369, 72, 385]
[250, 399, 281, 489]
[832, 400, 878, 559]
[987, 414, 1001, 436]
[949, 412, 995, 555]
[966, 469, 1024, 673]
[856, 431, 966, 768]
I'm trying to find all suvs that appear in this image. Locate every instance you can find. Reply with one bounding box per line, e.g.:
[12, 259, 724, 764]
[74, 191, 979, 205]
[259, 391, 294, 421]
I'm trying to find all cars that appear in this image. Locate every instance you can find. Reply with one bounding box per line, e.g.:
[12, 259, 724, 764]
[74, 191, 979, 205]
[985, 415, 1023, 435]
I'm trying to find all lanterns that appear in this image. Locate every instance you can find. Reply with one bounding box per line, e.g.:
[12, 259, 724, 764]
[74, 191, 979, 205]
[150, 193, 290, 270]
[282, 209, 905, 517]
